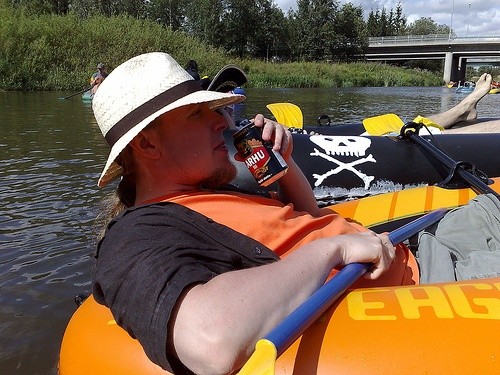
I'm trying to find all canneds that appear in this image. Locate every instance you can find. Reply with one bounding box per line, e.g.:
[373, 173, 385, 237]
[232, 122, 290, 187]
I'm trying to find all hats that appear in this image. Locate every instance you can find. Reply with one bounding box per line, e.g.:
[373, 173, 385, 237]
[96, 63, 104, 69]
[199, 63, 247, 92]
[91, 53, 247, 188]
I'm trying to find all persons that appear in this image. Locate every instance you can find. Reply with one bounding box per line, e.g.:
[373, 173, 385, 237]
[90, 64, 109, 94]
[492, 80, 500, 87]
[93, 51, 500, 375]
[199, 71, 494, 135]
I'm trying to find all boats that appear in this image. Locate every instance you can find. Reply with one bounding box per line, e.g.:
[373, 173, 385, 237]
[59, 104, 500, 375]
[446, 79, 500, 95]
